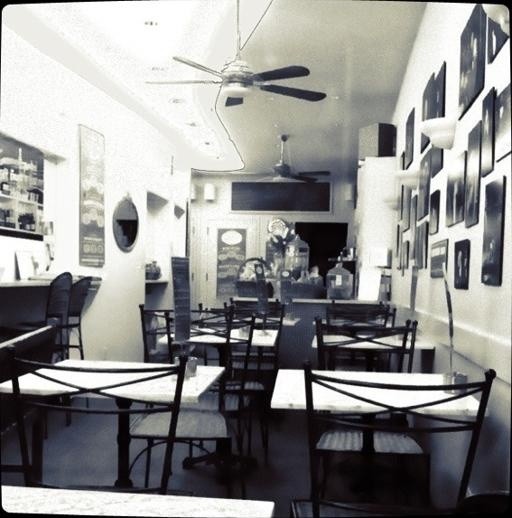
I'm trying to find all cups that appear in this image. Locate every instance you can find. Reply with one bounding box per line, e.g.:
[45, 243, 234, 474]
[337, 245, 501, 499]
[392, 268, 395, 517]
[174, 356, 198, 376]
[443, 372, 466, 394]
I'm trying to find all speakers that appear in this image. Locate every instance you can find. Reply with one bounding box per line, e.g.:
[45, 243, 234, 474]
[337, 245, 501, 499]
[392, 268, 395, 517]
[357, 121, 396, 158]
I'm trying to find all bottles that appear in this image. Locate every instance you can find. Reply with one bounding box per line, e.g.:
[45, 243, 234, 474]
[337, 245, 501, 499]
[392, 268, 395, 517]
[0, 165, 37, 233]
[145, 260, 162, 281]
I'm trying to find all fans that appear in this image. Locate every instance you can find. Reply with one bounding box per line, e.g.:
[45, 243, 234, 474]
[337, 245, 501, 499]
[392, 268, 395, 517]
[145, 1, 328, 107]
[237, 134, 330, 184]
